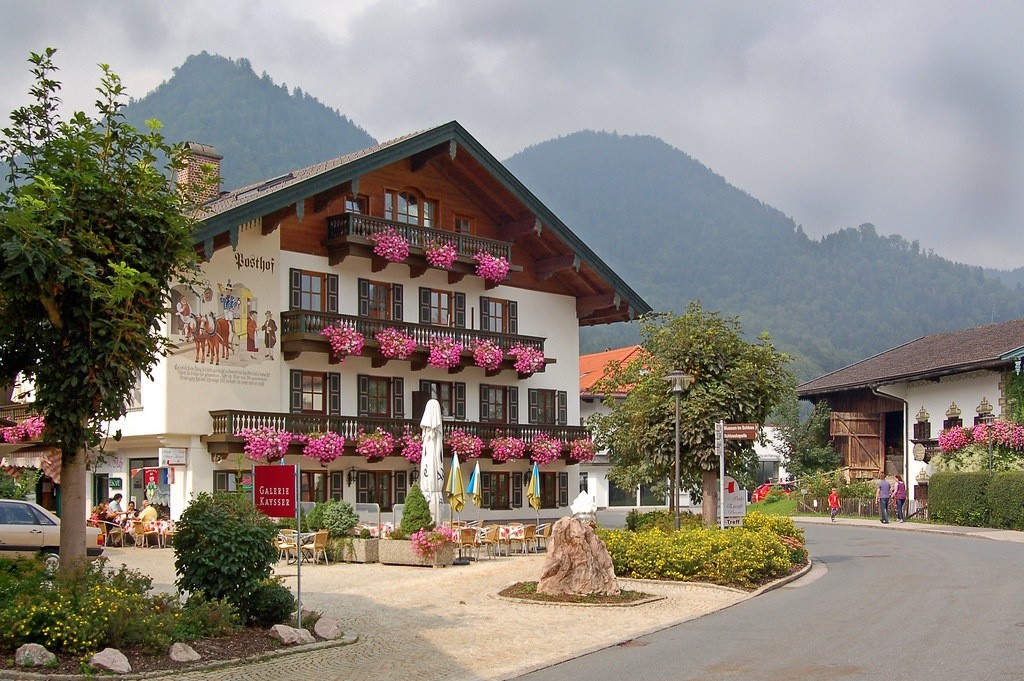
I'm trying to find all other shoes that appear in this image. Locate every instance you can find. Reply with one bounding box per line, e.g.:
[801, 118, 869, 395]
[114, 542, 122, 547]
[884, 521, 889, 523]
[896, 518, 900, 522]
[879, 519, 883, 523]
[899, 519, 903, 523]
[137, 543, 146, 548]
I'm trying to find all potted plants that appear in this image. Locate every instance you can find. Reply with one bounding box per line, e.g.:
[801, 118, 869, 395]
[319, 500, 378, 564]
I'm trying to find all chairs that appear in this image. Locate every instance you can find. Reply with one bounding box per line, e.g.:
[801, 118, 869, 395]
[273, 529, 329, 567]
[353, 520, 552, 562]
[87, 519, 179, 548]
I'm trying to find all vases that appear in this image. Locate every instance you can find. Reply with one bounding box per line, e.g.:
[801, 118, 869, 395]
[378, 539, 453, 568]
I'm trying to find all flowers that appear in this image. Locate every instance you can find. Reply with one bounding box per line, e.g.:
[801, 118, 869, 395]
[508, 340, 545, 379]
[472, 248, 509, 286]
[488, 428, 525, 466]
[469, 339, 503, 374]
[0, 416, 46, 444]
[422, 238, 456, 269]
[351, 426, 393, 461]
[529, 433, 562, 465]
[386, 483, 458, 557]
[321, 320, 363, 364]
[426, 334, 462, 370]
[442, 427, 484, 465]
[294, 429, 345, 467]
[366, 226, 411, 264]
[374, 327, 416, 360]
[568, 435, 597, 463]
[395, 424, 422, 464]
[234, 421, 291, 460]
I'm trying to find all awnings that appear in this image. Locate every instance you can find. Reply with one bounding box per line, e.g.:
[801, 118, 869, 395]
[0, 445, 62, 484]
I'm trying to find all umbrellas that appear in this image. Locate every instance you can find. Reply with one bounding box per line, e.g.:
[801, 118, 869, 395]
[446, 451, 466, 557]
[528, 462, 541, 546]
[420, 399, 445, 524]
[466, 461, 482, 520]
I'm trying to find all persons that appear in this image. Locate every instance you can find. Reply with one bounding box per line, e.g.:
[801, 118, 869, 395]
[828, 488, 841, 522]
[108, 494, 131, 521]
[98, 503, 126, 545]
[121, 501, 140, 543]
[891, 475, 906, 523]
[92, 507, 98, 527]
[129, 500, 157, 547]
[876, 475, 891, 523]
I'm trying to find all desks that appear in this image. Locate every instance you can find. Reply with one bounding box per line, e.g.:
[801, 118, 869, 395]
[285, 533, 315, 565]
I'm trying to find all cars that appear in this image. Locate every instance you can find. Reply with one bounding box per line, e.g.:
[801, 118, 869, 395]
[751, 482, 798, 503]
[0, 499, 106, 581]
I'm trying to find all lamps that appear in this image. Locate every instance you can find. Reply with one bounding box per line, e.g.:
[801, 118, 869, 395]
[346, 467, 357, 487]
[524, 468, 533, 486]
[409, 467, 419, 486]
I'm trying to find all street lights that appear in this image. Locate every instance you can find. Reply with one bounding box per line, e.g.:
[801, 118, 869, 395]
[978, 414, 1000, 475]
[660, 370, 693, 532]
